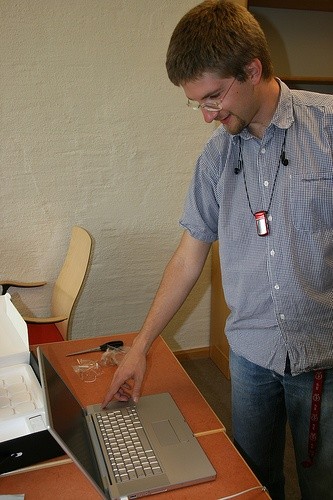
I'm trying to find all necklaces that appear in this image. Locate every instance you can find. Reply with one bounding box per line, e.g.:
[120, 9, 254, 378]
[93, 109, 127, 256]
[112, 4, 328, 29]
[238, 130, 286, 237]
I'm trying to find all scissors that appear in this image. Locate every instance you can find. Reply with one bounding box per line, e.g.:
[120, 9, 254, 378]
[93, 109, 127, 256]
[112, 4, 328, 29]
[65, 340, 123, 357]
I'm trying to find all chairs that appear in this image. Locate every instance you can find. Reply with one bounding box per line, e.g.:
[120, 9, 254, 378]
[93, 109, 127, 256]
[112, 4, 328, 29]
[0, 225, 95, 345]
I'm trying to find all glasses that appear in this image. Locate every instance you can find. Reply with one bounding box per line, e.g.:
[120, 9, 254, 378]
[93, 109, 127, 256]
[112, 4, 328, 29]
[186, 74, 236, 111]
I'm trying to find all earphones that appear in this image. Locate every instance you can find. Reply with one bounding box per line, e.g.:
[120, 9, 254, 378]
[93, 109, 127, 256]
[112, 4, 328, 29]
[281, 152, 289, 166]
[234, 160, 241, 175]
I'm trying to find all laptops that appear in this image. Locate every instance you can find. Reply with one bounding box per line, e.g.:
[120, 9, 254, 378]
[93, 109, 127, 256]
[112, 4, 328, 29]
[36, 347, 217, 500]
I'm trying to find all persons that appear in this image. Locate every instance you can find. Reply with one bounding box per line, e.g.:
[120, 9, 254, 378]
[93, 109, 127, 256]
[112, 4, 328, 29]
[102, 0, 333, 500]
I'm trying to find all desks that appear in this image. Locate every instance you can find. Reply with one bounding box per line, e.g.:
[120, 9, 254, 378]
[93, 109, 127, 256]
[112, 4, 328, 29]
[0, 331, 271, 500]
